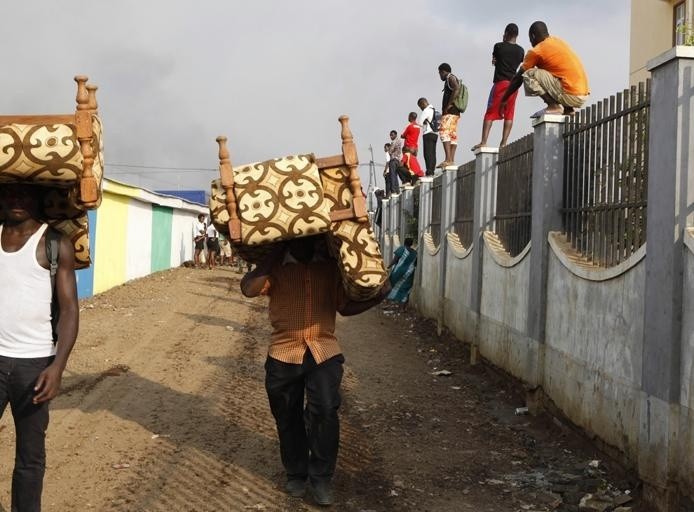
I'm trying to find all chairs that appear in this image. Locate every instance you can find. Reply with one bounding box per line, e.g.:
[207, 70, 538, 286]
[203, 113, 390, 305]
[0, 75, 105, 272]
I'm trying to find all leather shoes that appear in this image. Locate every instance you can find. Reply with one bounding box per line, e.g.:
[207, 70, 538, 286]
[285, 479, 308, 498]
[310, 481, 335, 505]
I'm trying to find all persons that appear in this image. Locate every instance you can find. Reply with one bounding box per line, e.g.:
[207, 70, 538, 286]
[193, 213, 238, 270]
[383, 112, 424, 199]
[241, 238, 393, 506]
[0, 183, 78, 512]
[498, 21, 591, 118]
[435, 63, 461, 168]
[471, 22, 525, 150]
[382, 238, 417, 313]
[418, 98, 438, 176]
